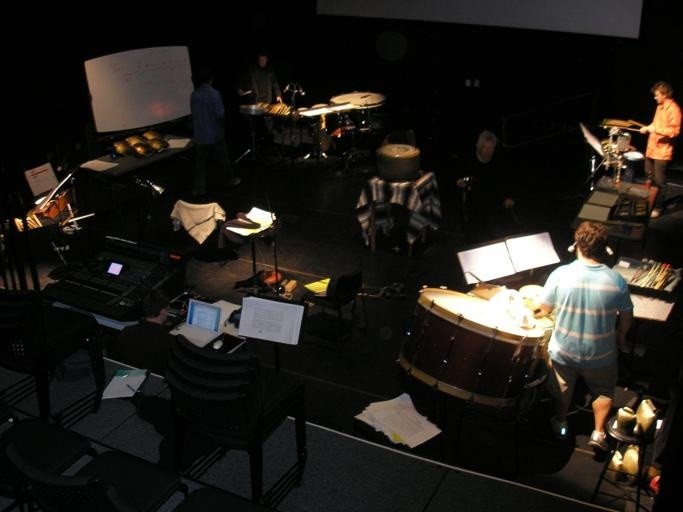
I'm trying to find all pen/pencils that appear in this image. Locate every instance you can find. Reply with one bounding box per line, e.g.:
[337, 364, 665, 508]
[361, 94, 372, 98]
[127, 384, 135, 392]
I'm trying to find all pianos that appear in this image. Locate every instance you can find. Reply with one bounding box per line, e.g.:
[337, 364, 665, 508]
[23, 171, 80, 233]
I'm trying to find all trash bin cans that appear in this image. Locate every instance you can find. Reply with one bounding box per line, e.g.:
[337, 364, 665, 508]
[85, 46, 194, 133]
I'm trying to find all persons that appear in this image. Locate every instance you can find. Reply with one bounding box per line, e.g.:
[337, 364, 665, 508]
[186, 69, 226, 196]
[236, 50, 284, 105]
[615, 255, 683, 290]
[540, 217, 634, 452]
[453, 130, 519, 241]
[117, 289, 174, 376]
[639, 80, 682, 218]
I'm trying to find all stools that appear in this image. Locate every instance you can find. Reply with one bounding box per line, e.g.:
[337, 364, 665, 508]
[591, 415, 655, 512]
[224, 221, 273, 298]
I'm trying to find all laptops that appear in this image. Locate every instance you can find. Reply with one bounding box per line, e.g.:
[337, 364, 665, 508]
[168, 295, 222, 348]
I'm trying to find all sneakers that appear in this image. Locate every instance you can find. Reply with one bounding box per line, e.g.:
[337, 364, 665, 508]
[551, 417, 568, 440]
[588, 431, 610, 452]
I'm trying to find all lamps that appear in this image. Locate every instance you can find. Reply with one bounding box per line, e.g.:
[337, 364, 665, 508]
[134, 175, 166, 201]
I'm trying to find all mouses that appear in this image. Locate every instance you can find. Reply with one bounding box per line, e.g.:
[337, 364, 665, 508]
[213, 339, 224, 350]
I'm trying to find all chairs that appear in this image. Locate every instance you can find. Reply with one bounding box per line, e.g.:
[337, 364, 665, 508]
[1, 290, 105, 425]
[301, 270, 369, 353]
[165, 334, 306, 507]
[0, 408, 285, 511]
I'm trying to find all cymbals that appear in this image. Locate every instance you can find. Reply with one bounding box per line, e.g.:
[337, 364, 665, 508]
[239, 104, 267, 117]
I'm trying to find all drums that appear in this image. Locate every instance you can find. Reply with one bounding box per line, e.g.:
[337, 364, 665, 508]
[470, 281, 556, 386]
[396, 285, 546, 408]
[623, 153, 646, 167]
[331, 92, 386, 130]
